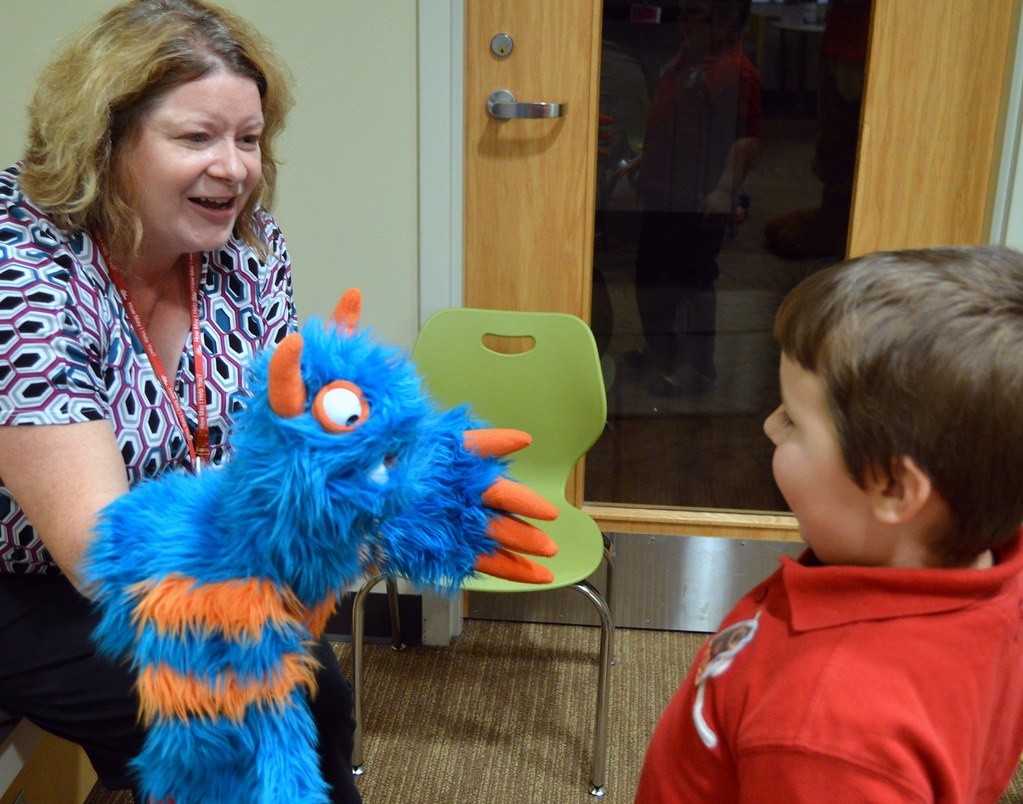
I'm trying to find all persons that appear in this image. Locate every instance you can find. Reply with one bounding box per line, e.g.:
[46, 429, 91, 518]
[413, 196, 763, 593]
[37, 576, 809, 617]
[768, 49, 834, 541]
[763, 0, 873, 252]
[0, 0, 365, 804]
[629, 246, 1020, 803]
[614, 0, 767, 394]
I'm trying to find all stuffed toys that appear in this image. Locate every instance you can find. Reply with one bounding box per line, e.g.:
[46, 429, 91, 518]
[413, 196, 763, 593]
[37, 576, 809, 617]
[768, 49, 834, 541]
[88, 289, 556, 804]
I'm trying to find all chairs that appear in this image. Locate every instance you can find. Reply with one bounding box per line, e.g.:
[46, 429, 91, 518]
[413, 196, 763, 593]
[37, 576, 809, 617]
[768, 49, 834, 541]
[349, 307, 619, 799]
